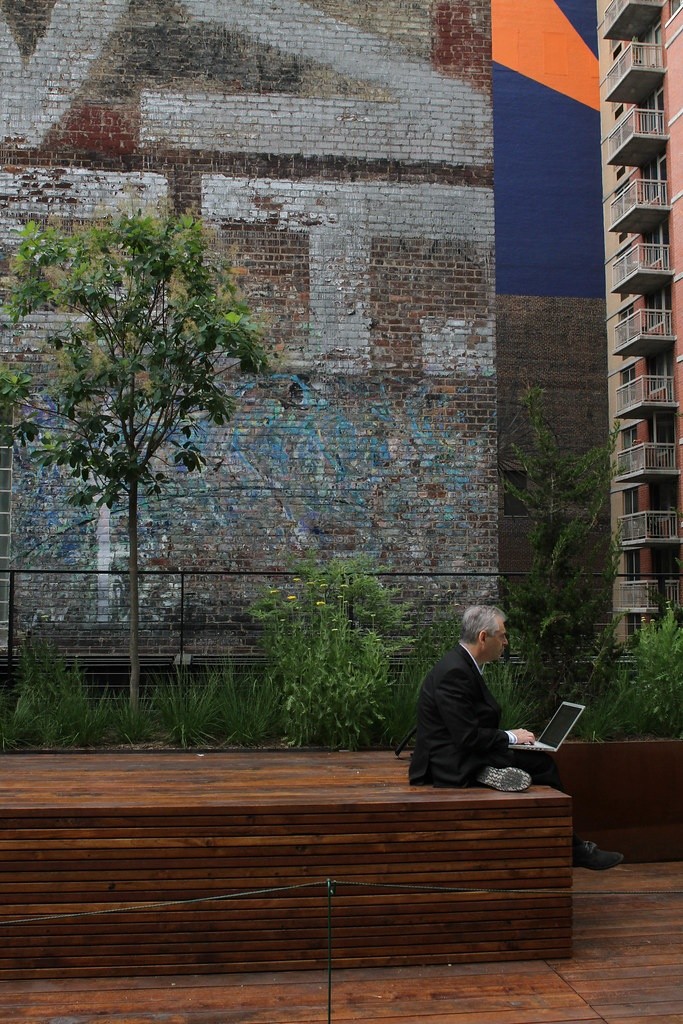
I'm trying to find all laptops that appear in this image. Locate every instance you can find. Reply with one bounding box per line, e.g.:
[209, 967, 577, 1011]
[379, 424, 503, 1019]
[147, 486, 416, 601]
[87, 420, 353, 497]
[506, 701, 586, 752]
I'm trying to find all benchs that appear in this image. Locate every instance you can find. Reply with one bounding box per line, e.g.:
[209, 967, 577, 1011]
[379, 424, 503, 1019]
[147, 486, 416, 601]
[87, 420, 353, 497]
[0, 751, 575, 980]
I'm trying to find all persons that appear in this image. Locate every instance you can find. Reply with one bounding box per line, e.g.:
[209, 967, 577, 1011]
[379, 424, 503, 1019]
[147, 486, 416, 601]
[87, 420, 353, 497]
[409, 605, 623, 870]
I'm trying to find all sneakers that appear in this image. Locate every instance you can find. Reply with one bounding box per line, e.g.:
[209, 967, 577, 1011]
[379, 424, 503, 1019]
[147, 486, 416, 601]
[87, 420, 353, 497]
[572, 831, 624, 871]
[474, 765, 533, 791]
